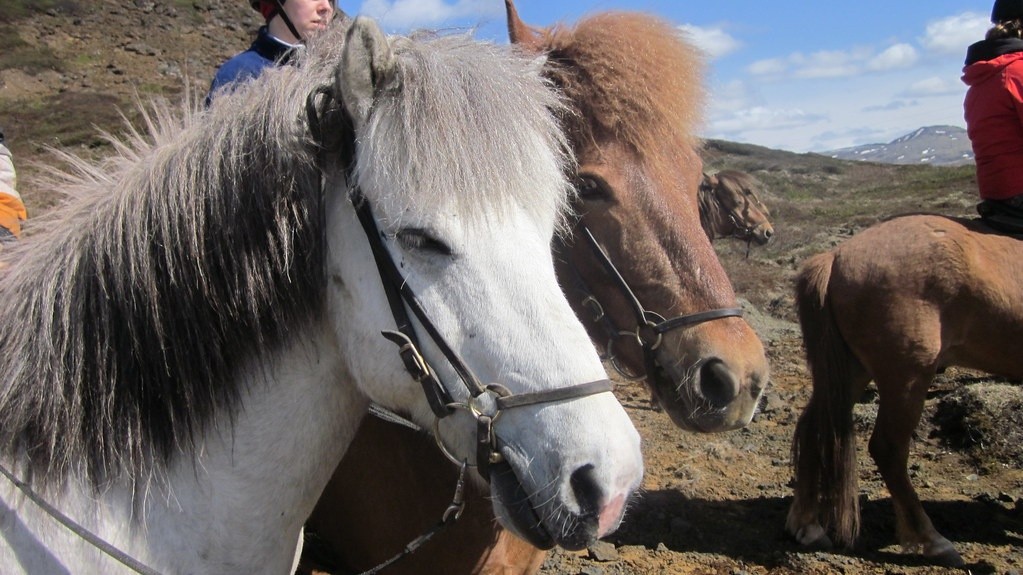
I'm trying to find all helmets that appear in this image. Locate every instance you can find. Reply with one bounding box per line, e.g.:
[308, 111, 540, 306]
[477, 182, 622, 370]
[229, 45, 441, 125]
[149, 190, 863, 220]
[991, 0, 1023, 23]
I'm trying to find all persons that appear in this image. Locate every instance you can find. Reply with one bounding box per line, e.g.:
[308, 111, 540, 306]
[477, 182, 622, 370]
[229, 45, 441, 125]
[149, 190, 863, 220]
[206, 0, 337, 106]
[0, 134, 26, 240]
[960, 0, 1023, 225]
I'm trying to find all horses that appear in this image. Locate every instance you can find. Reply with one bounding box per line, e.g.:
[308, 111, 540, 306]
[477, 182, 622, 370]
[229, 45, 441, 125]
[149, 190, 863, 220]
[0, 0, 1023, 575]
